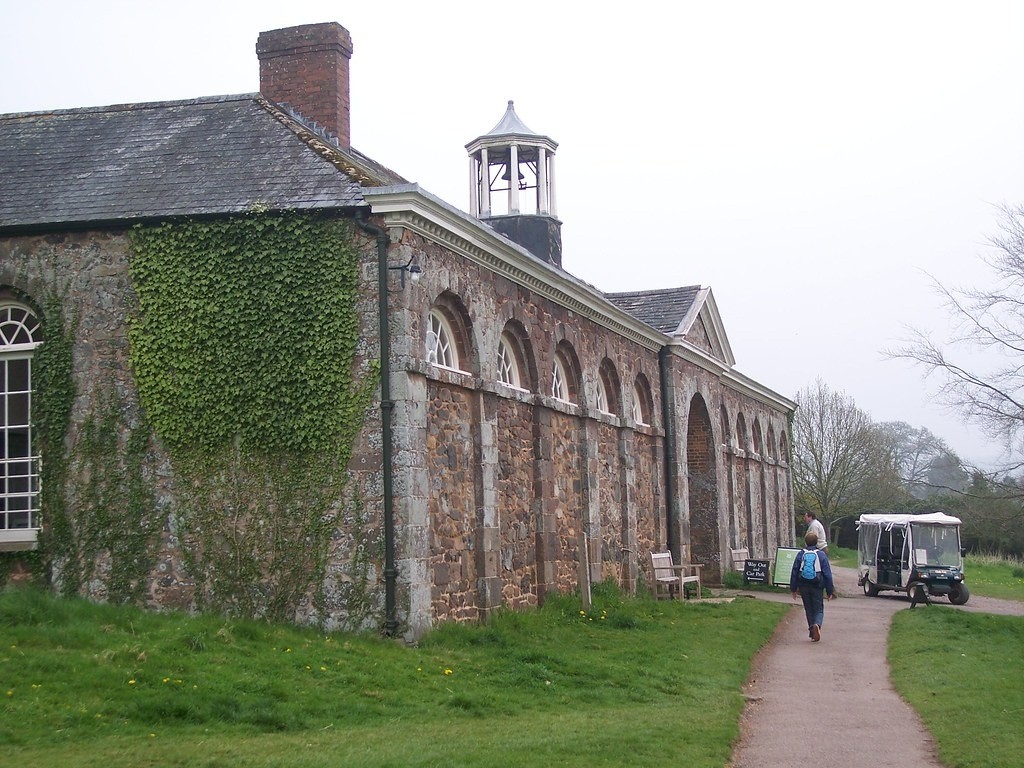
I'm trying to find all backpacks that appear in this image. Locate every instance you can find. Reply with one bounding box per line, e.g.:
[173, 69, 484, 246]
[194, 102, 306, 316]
[798, 549, 822, 584]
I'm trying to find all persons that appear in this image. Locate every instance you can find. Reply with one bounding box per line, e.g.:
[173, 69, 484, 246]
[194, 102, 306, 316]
[804, 511, 838, 598]
[790, 533, 833, 642]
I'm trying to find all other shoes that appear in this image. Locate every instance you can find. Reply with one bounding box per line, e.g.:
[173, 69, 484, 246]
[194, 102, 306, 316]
[811, 636, 816, 642]
[824, 590, 837, 599]
[813, 624, 820, 641]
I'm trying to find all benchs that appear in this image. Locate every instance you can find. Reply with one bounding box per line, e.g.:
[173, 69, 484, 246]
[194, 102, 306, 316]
[866, 545, 907, 583]
[729, 546, 772, 585]
[648, 550, 704, 602]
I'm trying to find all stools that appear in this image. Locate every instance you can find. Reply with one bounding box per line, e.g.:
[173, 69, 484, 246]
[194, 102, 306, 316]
[910, 583, 932, 608]
